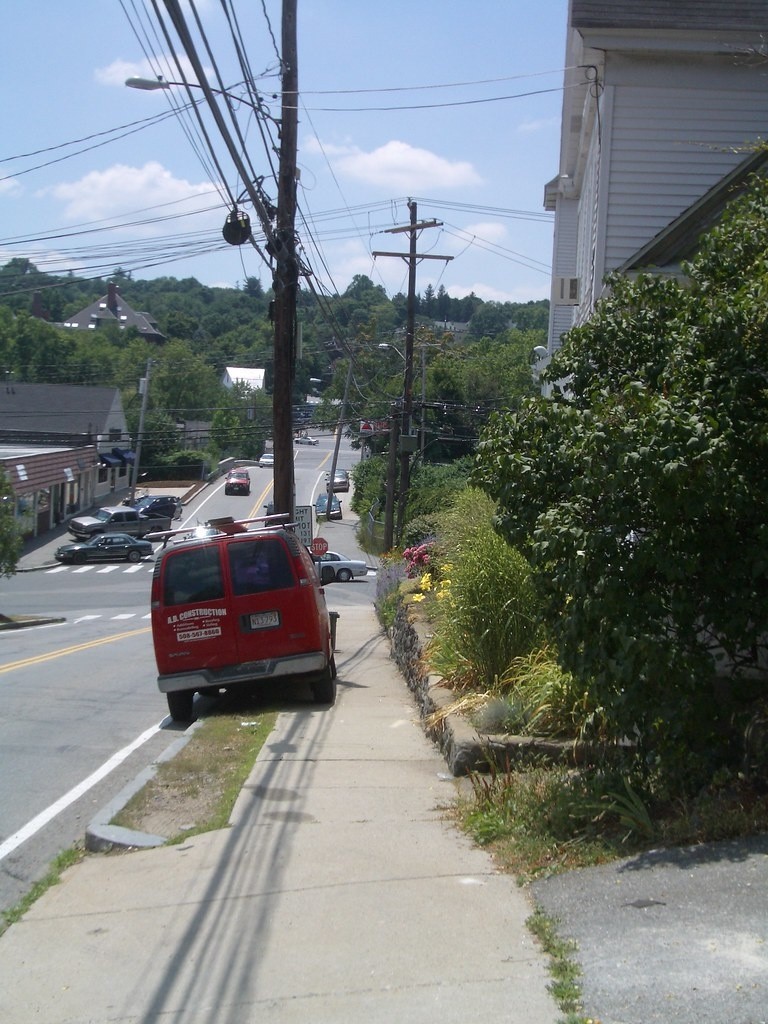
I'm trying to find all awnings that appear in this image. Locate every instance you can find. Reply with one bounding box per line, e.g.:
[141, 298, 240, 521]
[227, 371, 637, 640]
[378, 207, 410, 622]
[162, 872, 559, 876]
[100, 453, 127, 467]
[112, 449, 136, 463]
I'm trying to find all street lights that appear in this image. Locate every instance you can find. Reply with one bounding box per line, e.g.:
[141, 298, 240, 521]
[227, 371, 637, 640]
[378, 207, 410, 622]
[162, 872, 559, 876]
[126, 75, 300, 530]
[379, 342, 415, 549]
[309, 378, 349, 518]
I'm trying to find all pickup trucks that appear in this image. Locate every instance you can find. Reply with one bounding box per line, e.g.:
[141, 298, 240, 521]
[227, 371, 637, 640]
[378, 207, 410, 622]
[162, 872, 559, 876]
[68, 506, 171, 541]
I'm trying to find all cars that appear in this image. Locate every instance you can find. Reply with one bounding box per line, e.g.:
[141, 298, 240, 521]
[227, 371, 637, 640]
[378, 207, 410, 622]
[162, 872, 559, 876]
[295, 437, 320, 445]
[325, 473, 349, 493]
[225, 469, 251, 496]
[135, 495, 182, 520]
[309, 551, 369, 582]
[313, 494, 343, 522]
[326, 469, 349, 478]
[53, 532, 154, 565]
[259, 454, 274, 468]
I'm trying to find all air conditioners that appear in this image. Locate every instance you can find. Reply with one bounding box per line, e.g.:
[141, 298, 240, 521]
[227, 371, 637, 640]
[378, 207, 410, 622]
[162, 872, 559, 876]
[552, 277, 581, 306]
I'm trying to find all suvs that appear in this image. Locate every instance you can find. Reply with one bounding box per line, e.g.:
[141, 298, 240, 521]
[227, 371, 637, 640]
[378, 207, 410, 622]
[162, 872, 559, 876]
[143, 511, 338, 723]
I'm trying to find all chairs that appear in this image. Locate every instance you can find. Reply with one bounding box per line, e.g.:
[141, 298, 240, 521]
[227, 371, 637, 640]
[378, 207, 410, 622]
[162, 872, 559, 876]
[216, 549, 251, 589]
[265, 545, 296, 589]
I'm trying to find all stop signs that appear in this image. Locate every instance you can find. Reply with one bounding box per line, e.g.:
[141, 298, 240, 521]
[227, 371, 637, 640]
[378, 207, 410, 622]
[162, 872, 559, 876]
[311, 538, 328, 556]
